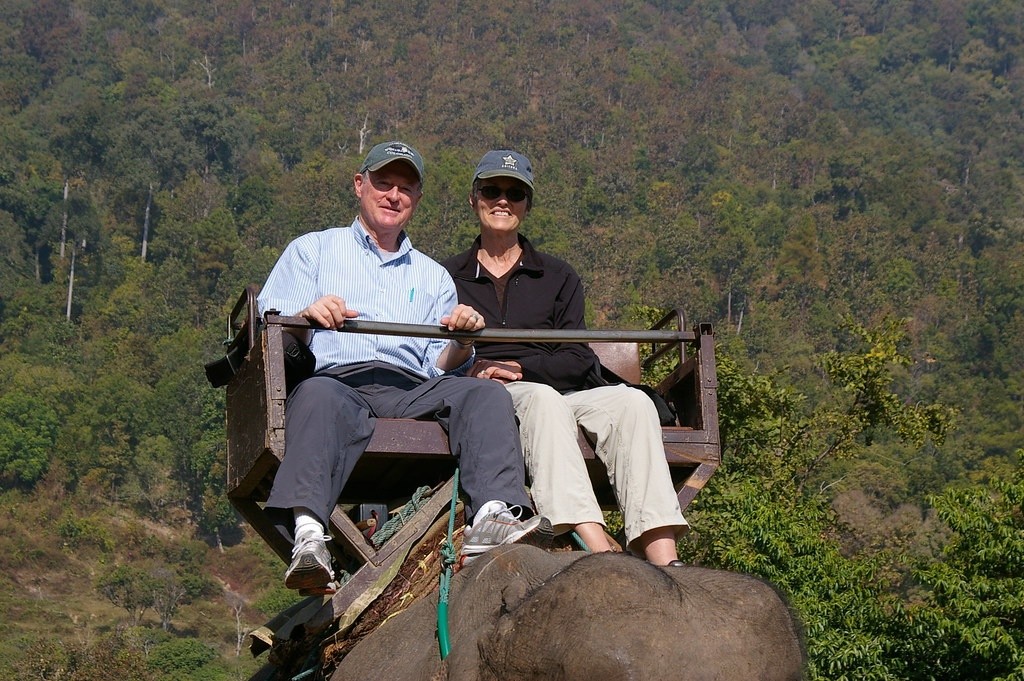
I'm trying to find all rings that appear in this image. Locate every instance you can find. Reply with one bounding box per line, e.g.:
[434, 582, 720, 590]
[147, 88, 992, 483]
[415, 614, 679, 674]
[470, 314, 478, 321]
[481, 370, 485, 375]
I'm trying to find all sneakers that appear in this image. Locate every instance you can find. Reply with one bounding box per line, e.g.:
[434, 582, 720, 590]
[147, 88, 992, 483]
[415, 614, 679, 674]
[285, 530, 334, 589]
[461, 503, 555, 566]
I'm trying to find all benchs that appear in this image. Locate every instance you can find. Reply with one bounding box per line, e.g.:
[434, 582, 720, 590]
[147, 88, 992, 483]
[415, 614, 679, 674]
[205, 288, 723, 655]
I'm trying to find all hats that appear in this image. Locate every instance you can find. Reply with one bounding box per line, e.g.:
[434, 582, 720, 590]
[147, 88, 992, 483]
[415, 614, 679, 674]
[360, 141, 425, 184]
[472, 151, 535, 192]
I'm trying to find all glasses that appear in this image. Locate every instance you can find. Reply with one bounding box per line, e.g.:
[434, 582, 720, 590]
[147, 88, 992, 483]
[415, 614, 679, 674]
[476, 185, 531, 203]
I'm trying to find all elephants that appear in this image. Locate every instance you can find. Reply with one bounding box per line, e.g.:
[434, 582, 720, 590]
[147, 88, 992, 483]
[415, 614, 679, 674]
[249, 543, 814, 681]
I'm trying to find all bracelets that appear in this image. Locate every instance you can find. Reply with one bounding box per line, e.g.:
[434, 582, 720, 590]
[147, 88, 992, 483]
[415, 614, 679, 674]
[451, 340, 474, 350]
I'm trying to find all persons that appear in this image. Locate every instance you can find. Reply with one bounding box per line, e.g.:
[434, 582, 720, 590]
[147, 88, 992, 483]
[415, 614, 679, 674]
[439, 148, 691, 566]
[254, 141, 554, 597]
